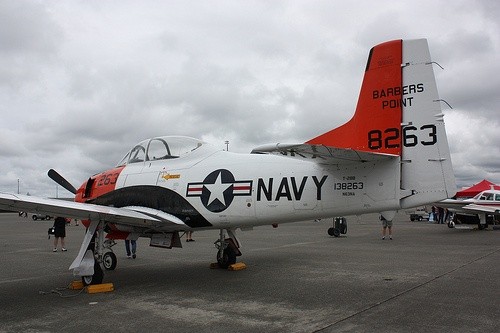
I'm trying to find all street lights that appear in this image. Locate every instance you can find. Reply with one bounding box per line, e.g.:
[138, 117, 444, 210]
[224, 140, 230, 152]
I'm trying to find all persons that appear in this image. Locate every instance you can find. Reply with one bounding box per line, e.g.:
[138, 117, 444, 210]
[431, 205, 445, 224]
[378, 215, 392, 240]
[125, 239, 136, 259]
[53, 215, 70, 252]
[185, 231, 195, 242]
[443, 208, 451, 224]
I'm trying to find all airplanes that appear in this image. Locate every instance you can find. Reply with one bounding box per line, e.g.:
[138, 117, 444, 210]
[0, 37, 458, 288]
[424, 184, 500, 230]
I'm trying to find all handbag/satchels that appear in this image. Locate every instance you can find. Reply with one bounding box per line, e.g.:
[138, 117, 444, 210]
[48, 226, 55, 239]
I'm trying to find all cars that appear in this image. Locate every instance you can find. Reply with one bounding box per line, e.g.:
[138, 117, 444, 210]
[32, 213, 54, 220]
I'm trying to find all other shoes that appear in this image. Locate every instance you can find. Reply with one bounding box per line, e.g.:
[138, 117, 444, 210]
[186, 239, 189, 242]
[389, 235, 392, 240]
[62, 248, 67, 251]
[189, 239, 194, 241]
[382, 237, 386, 240]
[133, 254, 136, 259]
[53, 248, 57, 252]
[128, 256, 131, 259]
